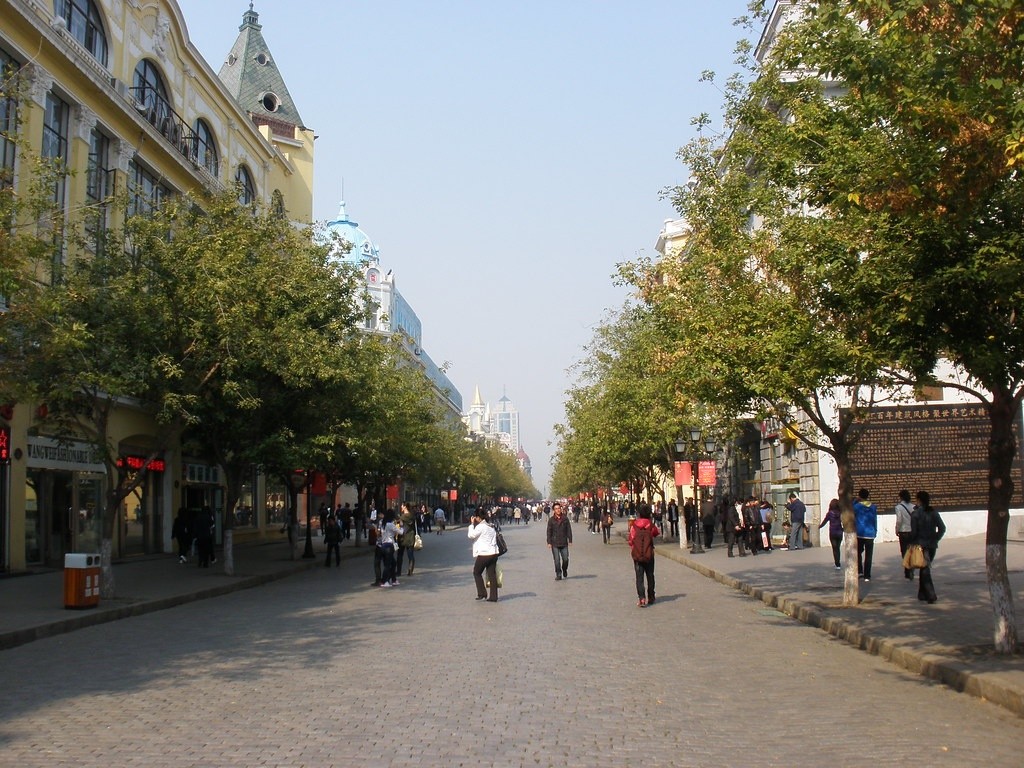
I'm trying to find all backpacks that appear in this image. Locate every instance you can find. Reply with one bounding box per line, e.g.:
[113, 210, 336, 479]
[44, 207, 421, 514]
[629, 521, 655, 563]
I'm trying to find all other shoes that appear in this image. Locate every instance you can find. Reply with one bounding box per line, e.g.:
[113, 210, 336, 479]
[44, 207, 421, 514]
[555, 576, 562, 581]
[864, 578, 870, 583]
[210, 558, 217, 565]
[834, 563, 841, 570]
[637, 596, 655, 607]
[858, 573, 863, 578]
[371, 580, 400, 588]
[563, 569, 567, 577]
[178, 555, 188, 565]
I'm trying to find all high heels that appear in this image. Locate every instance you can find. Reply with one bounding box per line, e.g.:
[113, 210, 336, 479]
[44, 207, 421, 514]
[475, 594, 487, 601]
[487, 597, 497, 602]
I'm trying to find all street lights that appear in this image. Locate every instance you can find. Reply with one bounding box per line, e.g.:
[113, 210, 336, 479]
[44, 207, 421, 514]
[671, 427, 717, 554]
[440, 475, 458, 526]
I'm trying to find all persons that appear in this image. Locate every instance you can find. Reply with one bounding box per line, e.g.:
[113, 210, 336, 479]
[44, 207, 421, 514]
[895, 490, 916, 580]
[324, 515, 341, 567]
[818, 499, 844, 569]
[910, 491, 946, 604]
[468, 509, 499, 602]
[443, 496, 647, 534]
[233, 504, 285, 527]
[651, 493, 806, 558]
[852, 488, 877, 582]
[601, 507, 613, 544]
[371, 502, 416, 587]
[547, 503, 572, 580]
[80, 503, 140, 536]
[628, 505, 660, 606]
[171, 506, 216, 568]
[280, 502, 447, 545]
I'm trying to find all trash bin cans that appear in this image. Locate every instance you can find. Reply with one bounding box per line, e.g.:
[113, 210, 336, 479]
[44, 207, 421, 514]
[63, 553, 103, 610]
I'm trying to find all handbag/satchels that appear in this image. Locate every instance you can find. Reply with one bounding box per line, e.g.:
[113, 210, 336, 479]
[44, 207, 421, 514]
[902, 545, 925, 570]
[492, 524, 508, 556]
[485, 562, 504, 589]
[414, 520, 423, 551]
